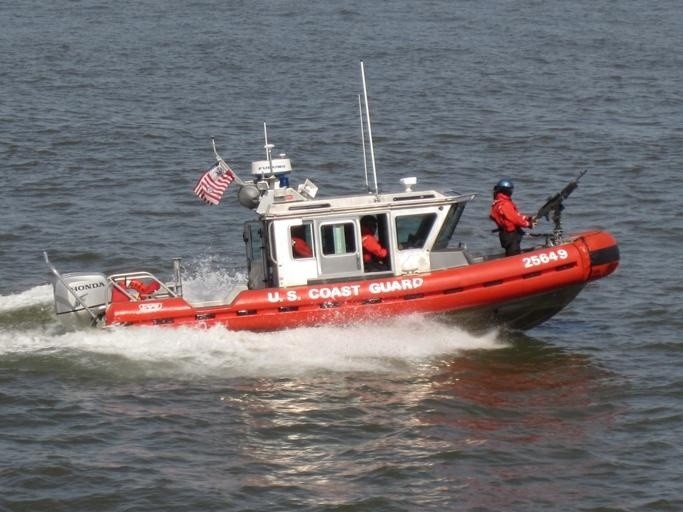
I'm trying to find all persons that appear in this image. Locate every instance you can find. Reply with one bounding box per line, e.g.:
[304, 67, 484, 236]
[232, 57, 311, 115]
[489, 179, 536, 257]
[290, 227, 312, 258]
[359, 215, 389, 271]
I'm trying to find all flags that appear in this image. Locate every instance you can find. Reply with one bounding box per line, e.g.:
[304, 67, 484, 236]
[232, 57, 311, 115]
[192, 159, 233, 207]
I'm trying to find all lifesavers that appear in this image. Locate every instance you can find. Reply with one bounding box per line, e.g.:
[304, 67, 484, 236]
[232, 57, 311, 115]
[116, 280, 146, 300]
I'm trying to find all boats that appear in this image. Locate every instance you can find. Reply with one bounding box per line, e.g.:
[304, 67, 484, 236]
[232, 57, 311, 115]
[40, 61, 621, 335]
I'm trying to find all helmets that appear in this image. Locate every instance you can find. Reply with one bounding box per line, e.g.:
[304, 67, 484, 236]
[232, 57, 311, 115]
[495, 179, 513, 195]
[360, 215, 378, 233]
